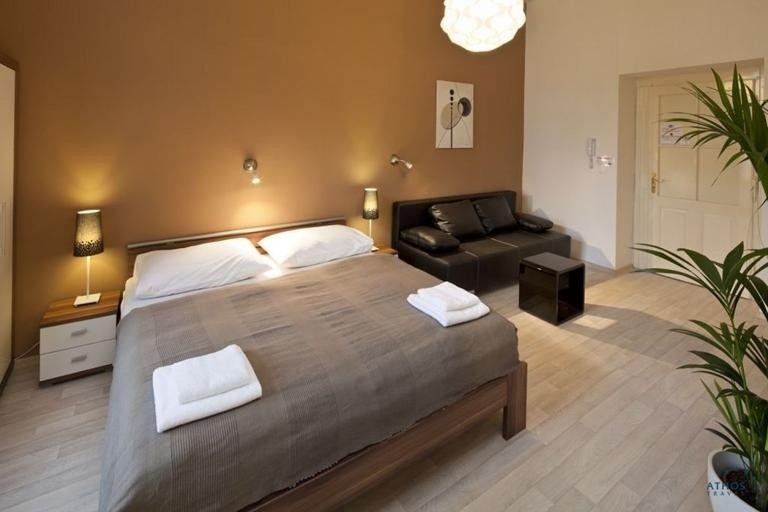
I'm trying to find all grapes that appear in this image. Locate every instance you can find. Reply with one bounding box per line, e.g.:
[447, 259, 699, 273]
[378, 245, 395, 254]
[38, 291, 120, 387]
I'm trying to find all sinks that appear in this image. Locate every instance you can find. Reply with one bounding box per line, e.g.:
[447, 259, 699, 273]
[393, 190, 571, 290]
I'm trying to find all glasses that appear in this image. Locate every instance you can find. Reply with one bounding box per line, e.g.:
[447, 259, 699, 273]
[72, 208, 103, 306]
[244, 159, 261, 185]
[390, 155, 414, 172]
[362, 188, 379, 251]
[440, 0, 525, 52]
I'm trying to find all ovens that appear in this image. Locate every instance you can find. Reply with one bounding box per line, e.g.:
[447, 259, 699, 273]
[100, 220, 528, 512]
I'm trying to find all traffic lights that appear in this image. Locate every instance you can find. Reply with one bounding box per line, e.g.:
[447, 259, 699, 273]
[514, 211, 555, 233]
[133, 238, 270, 300]
[430, 200, 485, 241]
[473, 196, 516, 234]
[399, 226, 459, 255]
[257, 224, 374, 269]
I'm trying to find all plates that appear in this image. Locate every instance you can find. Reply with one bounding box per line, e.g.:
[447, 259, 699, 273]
[0, 51, 19, 394]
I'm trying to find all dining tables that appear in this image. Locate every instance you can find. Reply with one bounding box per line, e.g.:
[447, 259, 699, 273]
[628, 63, 768, 511]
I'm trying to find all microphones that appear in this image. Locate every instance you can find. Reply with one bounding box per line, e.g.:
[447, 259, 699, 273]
[518, 252, 585, 326]
